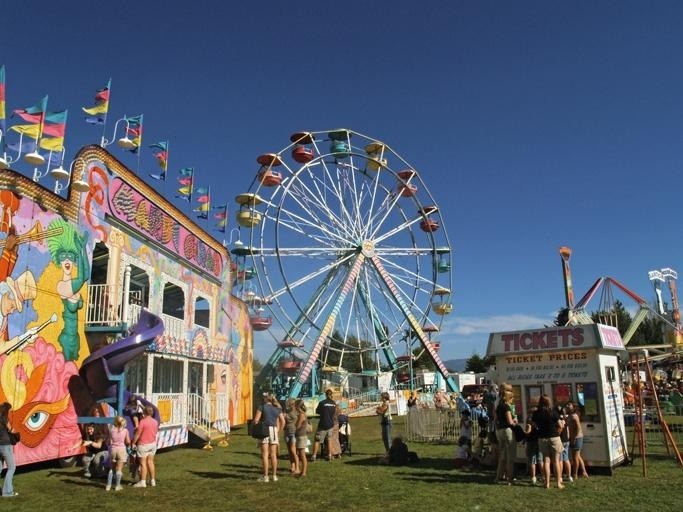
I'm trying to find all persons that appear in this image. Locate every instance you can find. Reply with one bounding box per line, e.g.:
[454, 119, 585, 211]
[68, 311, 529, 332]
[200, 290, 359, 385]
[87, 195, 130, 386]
[79, 424, 107, 478]
[0, 401, 21, 500]
[620, 375, 682, 432]
[104, 416, 131, 493]
[122, 396, 145, 484]
[382, 437, 408, 466]
[131, 403, 159, 488]
[374, 392, 392, 459]
[407, 390, 418, 410]
[249, 385, 352, 484]
[431, 379, 590, 491]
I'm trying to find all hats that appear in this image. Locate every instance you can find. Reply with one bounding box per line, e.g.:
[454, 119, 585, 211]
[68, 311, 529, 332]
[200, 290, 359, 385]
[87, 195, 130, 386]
[262, 392, 274, 400]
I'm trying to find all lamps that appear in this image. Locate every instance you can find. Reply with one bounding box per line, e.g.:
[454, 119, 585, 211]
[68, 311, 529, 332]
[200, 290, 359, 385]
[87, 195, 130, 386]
[238, 279, 264, 314]
[54, 157, 89, 198]
[33, 144, 68, 186]
[222, 227, 242, 248]
[0, 125, 44, 168]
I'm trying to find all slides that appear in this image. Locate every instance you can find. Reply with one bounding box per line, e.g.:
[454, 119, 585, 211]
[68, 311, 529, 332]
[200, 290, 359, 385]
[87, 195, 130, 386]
[79, 309, 164, 474]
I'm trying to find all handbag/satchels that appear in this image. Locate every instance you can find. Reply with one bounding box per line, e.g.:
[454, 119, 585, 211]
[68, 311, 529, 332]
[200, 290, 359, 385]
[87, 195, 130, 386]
[306, 419, 313, 434]
[513, 424, 524, 442]
[247, 419, 269, 438]
[8, 431, 20, 447]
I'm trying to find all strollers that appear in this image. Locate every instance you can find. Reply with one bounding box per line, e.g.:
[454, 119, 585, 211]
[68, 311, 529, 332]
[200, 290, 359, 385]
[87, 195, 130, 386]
[321, 412, 351, 460]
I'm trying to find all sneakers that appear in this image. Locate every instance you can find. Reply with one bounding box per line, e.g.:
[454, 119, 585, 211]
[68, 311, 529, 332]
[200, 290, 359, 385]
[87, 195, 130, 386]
[272, 476, 279, 482]
[84, 472, 92, 478]
[307, 455, 317, 461]
[3, 491, 18, 497]
[105, 484, 112, 492]
[288, 468, 306, 478]
[496, 476, 517, 486]
[255, 475, 270, 484]
[148, 480, 156, 487]
[531, 475, 590, 491]
[114, 486, 123, 492]
[132, 480, 147, 488]
